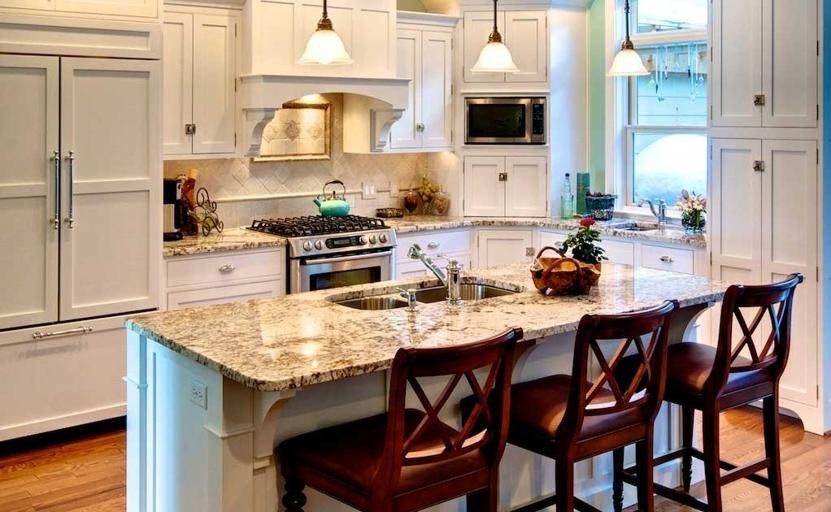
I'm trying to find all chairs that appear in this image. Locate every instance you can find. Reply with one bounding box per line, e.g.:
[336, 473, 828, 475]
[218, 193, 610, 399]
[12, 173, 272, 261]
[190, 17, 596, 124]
[456, 297, 679, 512]
[275, 323, 524, 511]
[607, 271, 804, 512]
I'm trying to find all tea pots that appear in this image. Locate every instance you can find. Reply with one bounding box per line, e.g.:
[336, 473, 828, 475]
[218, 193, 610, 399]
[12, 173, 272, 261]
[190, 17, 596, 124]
[310, 177, 350, 219]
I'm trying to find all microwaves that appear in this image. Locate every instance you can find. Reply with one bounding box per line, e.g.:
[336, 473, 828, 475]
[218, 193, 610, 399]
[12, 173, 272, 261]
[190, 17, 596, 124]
[461, 95, 547, 146]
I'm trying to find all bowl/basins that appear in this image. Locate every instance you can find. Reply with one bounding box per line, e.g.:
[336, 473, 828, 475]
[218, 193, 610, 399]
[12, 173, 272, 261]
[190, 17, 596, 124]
[584, 193, 618, 222]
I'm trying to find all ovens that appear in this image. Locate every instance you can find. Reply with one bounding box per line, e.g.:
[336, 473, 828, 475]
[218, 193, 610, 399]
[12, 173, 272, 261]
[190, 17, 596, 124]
[287, 246, 398, 291]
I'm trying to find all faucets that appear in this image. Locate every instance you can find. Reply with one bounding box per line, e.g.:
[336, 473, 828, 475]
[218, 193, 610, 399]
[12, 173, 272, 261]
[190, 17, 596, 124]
[637, 198, 668, 223]
[395, 286, 418, 311]
[407, 242, 461, 306]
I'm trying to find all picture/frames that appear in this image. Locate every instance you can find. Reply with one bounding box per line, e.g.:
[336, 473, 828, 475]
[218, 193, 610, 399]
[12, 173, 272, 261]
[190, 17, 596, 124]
[250, 101, 332, 163]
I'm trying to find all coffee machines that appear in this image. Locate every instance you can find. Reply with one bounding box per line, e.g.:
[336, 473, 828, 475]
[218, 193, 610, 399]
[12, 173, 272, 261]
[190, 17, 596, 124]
[163, 169, 201, 242]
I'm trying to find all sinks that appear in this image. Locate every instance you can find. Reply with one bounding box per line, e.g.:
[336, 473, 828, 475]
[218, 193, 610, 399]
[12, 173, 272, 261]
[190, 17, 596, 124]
[324, 285, 419, 311]
[601, 222, 676, 232]
[398, 276, 527, 305]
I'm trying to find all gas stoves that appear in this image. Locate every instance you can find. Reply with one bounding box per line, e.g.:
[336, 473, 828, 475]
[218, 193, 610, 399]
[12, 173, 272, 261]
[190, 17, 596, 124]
[241, 212, 401, 259]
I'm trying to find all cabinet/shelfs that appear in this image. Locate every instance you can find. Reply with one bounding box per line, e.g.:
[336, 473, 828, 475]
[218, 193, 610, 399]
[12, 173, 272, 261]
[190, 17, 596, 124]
[342, 10, 464, 155]
[430, 147, 590, 219]
[1, 317, 127, 440]
[707, 131, 831, 437]
[455, 0, 589, 88]
[536, 227, 637, 266]
[476, 224, 536, 270]
[707, 1, 830, 132]
[637, 238, 706, 345]
[1, 53, 163, 334]
[164, 246, 288, 314]
[395, 225, 475, 280]
[163, 0, 242, 161]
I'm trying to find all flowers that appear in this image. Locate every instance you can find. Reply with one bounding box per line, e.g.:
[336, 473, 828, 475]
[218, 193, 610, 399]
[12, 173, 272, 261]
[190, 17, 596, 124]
[559, 217, 609, 264]
[676, 189, 707, 224]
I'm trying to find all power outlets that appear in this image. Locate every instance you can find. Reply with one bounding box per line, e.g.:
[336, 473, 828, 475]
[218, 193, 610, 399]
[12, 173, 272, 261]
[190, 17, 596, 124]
[188, 377, 208, 410]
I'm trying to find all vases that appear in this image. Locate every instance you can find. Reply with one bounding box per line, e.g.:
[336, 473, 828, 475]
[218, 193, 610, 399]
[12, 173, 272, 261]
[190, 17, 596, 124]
[682, 211, 706, 231]
[588, 262, 602, 287]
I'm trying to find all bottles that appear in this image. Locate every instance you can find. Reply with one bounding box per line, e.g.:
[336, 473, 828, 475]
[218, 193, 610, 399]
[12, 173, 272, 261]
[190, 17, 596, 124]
[560, 171, 590, 221]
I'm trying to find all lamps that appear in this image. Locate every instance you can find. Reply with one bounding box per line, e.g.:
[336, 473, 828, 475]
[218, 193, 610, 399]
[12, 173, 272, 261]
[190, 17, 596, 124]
[604, 1, 651, 78]
[298, 0, 354, 68]
[469, 0, 521, 74]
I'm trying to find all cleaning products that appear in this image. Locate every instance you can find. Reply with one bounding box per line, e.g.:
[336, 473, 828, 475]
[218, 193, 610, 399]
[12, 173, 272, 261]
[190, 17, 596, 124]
[560, 172, 574, 220]
